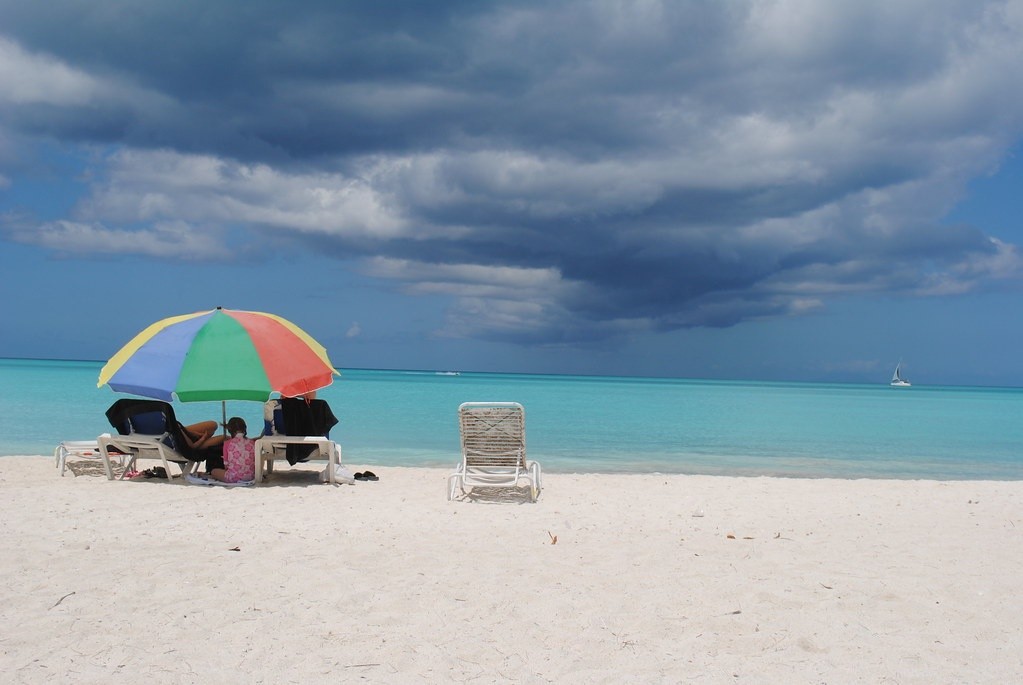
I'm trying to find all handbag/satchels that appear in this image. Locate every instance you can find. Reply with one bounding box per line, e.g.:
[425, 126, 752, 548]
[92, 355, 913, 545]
[319, 464, 354, 484]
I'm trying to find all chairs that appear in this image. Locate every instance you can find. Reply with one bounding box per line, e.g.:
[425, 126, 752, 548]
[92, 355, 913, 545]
[254, 398, 342, 484]
[447, 401, 541, 503]
[98, 399, 223, 481]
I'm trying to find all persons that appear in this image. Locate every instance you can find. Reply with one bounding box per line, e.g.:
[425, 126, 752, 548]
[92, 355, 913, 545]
[177, 421, 229, 450]
[212, 417, 255, 482]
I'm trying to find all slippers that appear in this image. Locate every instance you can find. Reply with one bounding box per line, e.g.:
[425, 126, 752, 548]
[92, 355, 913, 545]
[354, 471, 379, 481]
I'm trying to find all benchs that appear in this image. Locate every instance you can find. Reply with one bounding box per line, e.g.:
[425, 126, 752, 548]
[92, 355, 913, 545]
[55, 440, 133, 477]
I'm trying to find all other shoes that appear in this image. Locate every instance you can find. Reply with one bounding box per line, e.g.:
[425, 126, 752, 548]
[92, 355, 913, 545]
[142, 466, 168, 478]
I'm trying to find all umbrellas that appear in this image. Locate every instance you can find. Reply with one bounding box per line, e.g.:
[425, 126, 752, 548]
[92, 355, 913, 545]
[98, 306, 340, 438]
